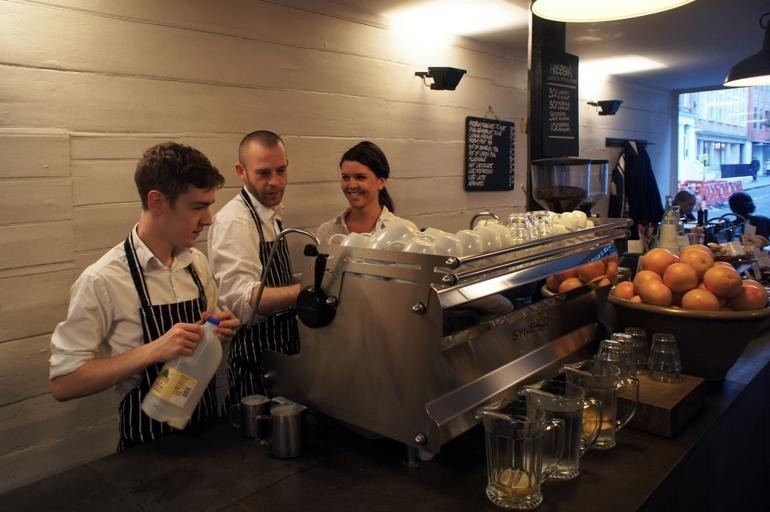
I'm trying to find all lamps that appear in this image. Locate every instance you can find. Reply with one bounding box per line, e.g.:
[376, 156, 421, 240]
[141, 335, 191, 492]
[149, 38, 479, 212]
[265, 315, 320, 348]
[587, 98, 624, 116]
[415, 64, 466, 95]
[721, 11, 770, 89]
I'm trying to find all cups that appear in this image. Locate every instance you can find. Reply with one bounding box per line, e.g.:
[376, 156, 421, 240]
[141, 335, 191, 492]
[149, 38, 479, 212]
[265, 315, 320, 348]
[324, 216, 512, 287]
[596, 326, 686, 388]
[559, 357, 639, 454]
[254, 404, 310, 459]
[687, 233, 707, 247]
[509, 207, 596, 263]
[658, 222, 679, 256]
[516, 378, 603, 480]
[227, 395, 272, 440]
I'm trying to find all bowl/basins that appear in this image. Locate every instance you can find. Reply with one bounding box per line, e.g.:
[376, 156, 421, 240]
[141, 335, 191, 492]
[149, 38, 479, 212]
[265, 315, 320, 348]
[607, 286, 769, 385]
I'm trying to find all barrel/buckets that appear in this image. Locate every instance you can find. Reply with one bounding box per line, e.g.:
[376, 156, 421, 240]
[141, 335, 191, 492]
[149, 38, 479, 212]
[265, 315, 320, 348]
[141, 315, 224, 430]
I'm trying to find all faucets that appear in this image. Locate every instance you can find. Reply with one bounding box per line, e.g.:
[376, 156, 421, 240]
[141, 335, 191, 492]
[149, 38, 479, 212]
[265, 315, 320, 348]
[705, 217, 735, 241]
[718, 213, 746, 222]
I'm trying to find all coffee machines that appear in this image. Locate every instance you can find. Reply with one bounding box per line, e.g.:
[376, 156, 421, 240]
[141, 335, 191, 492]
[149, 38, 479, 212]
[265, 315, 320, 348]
[577, 159, 609, 218]
[528, 156, 589, 213]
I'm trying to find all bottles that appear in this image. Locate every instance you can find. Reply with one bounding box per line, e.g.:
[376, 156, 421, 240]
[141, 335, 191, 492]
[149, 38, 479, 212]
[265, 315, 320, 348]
[697, 200, 708, 226]
[662, 195, 679, 234]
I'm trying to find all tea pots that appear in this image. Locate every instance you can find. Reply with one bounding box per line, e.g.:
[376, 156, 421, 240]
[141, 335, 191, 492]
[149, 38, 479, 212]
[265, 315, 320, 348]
[472, 398, 568, 511]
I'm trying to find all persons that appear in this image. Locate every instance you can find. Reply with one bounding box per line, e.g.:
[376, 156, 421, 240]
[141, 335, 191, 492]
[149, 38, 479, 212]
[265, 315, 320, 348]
[315, 141, 422, 245]
[749, 156, 760, 181]
[206, 129, 301, 421]
[47, 140, 242, 454]
[764, 159, 770, 175]
[728, 191, 770, 238]
[671, 190, 696, 225]
